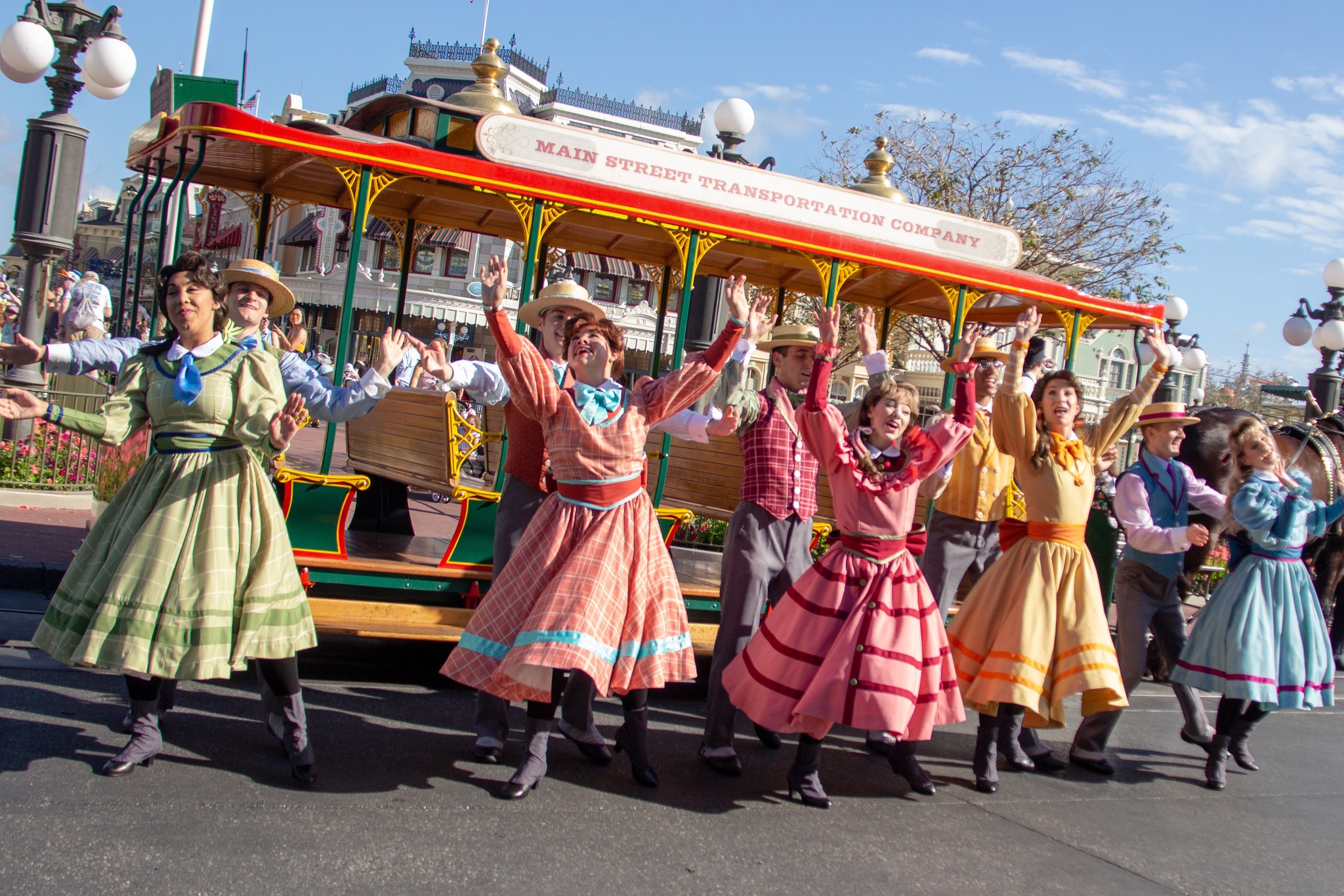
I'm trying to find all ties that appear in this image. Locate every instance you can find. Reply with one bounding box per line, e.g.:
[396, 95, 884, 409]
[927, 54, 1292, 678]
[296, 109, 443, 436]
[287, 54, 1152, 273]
[1167, 463, 1177, 505]
[173, 355, 203, 407]
[552, 366, 566, 389]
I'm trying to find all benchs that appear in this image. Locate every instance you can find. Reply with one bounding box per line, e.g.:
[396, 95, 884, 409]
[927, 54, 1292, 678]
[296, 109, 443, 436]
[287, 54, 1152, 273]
[271, 408, 371, 560]
[646, 421, 831, 554]
[345, 388, 508, 573]
[483, 403, 694, 551]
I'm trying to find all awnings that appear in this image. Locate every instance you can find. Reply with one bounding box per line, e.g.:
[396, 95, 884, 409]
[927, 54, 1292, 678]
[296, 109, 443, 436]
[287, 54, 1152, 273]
[194, 222, 242, 249]
[136, 202, 160, 215]
[540, 251, 681, 287]
[103, 246, 125, 267]
[362, 214, 471, 253]
[127, 257, 155, 271]
[278, 209, 351, 248]
[79, 247, 98, 264]
[114, 252, 135, 269]
[73, 254, 80, 265]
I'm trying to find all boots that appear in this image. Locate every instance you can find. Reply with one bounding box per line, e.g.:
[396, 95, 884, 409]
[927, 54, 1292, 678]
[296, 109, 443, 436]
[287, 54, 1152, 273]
[1227, 714, 1260, 770]
[1205, 735, 1231, 790]
[98, 688, 1070, 809]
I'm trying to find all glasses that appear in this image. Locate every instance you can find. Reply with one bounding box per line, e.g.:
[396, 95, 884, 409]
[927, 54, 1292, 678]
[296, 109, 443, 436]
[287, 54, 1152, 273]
[0, 287, 6, 290]
[974, 359, 1004, 371]
[289, 314, 301, 318]
[61, 278, 73, 282]
[262, 317, 271, 321]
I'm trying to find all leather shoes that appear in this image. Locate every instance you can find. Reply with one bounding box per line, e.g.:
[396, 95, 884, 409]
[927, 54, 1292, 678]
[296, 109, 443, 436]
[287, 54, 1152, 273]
[1181, 727, 1231, 757]
[1070, 751, 1116, 776]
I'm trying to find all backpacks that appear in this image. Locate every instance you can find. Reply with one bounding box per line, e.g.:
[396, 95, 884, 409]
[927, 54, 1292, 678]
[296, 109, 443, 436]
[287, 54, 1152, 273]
[75, 283, 97, 329]
[309, 353, 331, 371]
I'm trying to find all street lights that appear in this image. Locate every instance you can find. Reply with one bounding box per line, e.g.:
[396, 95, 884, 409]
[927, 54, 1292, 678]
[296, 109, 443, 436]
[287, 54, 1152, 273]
[1132, 297, 1208, 417]
[666, 98, 777, 357]
[1282, 260, 1344, 422]
[1, 1, 137, 442]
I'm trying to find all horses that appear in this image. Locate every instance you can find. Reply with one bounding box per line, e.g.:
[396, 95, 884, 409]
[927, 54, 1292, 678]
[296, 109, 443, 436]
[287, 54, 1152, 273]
[1146, 405, 1344, 684]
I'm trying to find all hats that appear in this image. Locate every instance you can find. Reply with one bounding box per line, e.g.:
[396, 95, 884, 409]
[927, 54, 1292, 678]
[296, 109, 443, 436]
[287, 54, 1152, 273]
[518, 282, 606, 330]
[1130, 402, 1200, 429]
[755, 325, 822, 353]
[83, 271, 100, 282]
[344, 363, 355, 371]
[940, 337, 1009, 373]
[6, 305, 20, 314]
[315, 345, 323, 351]
[214, 258, 295, 317]
[58, 269, 80, 282]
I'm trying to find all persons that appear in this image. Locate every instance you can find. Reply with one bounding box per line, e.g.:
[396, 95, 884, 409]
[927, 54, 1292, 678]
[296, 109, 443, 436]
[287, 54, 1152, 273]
[438, 255, 748, 806]
[0, 258, 410, 737]
[408, 277, 739, 764]
[696, 294, 898, 776]
[0, 269, 149, 377]
[1069, 401, 1230, 778]
[866, 339, 1116, 760]
[1171, 417, 1344, 791]
[394, 331, 479, 465]
[949, 306, 1171, 793]
[0, 250, 320, 788]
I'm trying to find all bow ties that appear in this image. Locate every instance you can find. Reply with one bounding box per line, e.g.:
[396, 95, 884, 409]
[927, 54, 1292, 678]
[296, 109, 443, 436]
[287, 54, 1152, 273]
[575, 384, 622, 425]
[235, 334, 258, 351]
[785, 389, 807, 410]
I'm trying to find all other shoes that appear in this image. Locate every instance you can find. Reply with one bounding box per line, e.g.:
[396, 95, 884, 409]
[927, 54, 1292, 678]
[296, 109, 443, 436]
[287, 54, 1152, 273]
[311, 421, 320, 427]
